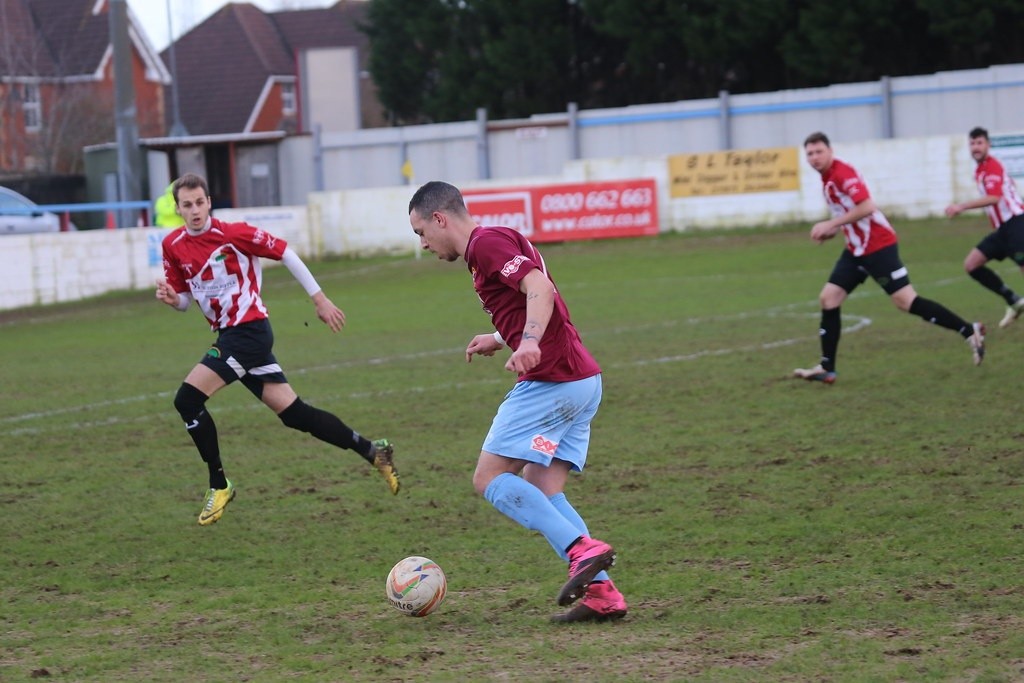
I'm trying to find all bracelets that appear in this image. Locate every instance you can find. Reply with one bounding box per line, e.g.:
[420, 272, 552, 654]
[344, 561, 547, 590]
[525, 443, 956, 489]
[493, 332, 506, 345]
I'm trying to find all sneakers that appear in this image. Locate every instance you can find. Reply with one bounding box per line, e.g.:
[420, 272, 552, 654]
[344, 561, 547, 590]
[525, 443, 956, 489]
[372, 439, 400, 495]
[551, 580, 627, 624]
[197, 477, 236, 525]
[963, 322, 987, 366]
[557, 536, 616, 606]
[999, 297, 1024, 328]
[796, 365, 835, 386]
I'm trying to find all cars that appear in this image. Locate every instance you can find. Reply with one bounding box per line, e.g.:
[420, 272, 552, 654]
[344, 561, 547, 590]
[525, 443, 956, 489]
[0, 186, 78, 234]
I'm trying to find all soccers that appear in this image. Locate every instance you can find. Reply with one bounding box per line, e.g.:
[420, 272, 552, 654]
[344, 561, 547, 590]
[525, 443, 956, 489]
[385, 555, 448, 617]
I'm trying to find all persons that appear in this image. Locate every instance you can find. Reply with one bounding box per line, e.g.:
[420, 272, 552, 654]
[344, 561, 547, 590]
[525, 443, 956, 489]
[408, 182, 628, 621]
[153, 172, 400, 525]
[945, 126, 1024, 327]
[794, 133, 984, 383]
